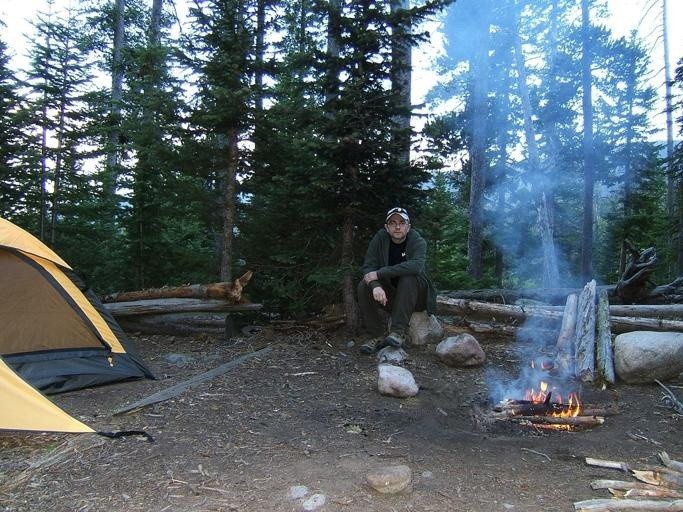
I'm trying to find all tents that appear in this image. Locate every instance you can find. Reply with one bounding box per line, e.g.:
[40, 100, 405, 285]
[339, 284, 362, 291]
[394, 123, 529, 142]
[0, 216, 160, 443]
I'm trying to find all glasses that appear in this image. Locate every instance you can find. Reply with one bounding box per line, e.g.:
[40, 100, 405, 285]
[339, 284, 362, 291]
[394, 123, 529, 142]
[389, 221, 406, 227]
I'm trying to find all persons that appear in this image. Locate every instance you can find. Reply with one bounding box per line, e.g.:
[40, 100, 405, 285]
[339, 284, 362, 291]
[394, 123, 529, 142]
[357, 207, 437, 355]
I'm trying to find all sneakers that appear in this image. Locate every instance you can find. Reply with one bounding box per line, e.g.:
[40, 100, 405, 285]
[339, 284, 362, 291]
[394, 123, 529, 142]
[360, 336, 386, 354]
[385, 332, 406, 348]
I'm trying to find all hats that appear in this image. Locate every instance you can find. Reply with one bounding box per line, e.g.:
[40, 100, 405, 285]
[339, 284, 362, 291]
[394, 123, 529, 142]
[386, 207, 410, 221]
